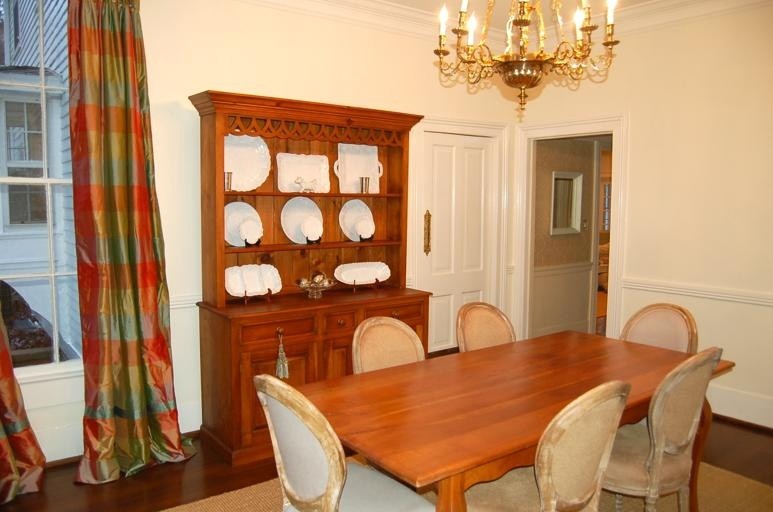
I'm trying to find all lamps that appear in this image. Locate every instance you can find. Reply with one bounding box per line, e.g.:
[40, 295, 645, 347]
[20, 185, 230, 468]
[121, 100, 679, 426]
[433, 0, 619, 111]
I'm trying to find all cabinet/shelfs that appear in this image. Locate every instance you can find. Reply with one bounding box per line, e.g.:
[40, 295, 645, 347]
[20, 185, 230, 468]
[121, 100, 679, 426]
[188, 88, 434, 469]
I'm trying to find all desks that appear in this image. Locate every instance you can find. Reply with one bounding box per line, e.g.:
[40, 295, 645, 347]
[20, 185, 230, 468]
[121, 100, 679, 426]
[294, 328, 735, 512]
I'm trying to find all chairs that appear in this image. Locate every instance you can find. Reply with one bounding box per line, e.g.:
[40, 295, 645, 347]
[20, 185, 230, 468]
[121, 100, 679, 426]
[603, 347, 724, 511]
[455, 301, 518, 352]
[619, 303, 698, 356]
[353, 315, 426, 374]
[251, 373, 439, 512]
[463, 380, 635, 512]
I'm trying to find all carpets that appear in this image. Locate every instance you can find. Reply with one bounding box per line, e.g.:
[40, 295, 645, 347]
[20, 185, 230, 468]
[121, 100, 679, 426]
[168, 452, 773, 512]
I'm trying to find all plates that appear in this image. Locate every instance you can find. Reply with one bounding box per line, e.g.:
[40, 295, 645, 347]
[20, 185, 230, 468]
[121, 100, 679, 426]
[221, 129, 391, 299]
